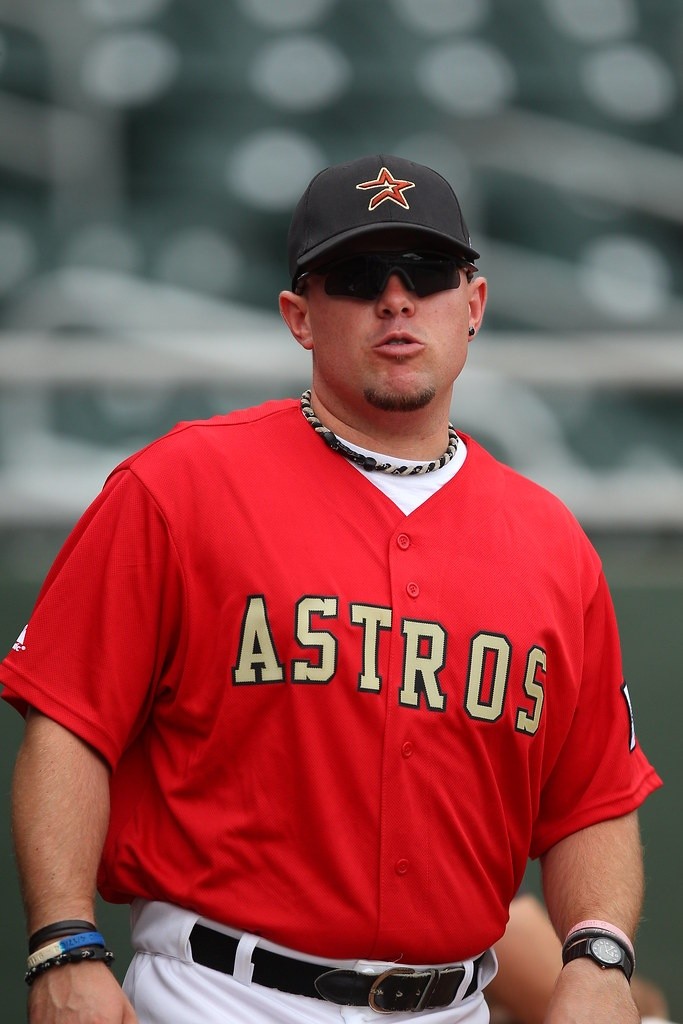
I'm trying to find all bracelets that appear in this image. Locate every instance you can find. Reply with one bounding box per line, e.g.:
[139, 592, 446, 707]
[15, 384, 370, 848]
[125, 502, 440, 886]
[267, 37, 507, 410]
[24, 920, 113, 986]
[562, 920, 636, 968]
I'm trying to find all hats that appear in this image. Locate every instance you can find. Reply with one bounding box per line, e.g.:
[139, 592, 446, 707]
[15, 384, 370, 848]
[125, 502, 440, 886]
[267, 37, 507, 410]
[289, 154, 481, 292]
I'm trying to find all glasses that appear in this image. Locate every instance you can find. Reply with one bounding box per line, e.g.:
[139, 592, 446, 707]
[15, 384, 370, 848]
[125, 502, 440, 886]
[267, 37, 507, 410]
[295, 238, 478, 301]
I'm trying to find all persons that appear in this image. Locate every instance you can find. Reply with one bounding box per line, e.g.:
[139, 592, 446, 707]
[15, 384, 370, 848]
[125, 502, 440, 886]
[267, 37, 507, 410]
[479, 896, 669, 1024]
[0, 153, 664, 1024]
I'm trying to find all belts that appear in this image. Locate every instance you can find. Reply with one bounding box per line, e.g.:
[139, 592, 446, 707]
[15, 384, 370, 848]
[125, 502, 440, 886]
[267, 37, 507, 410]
[189, 924, 486, 1015]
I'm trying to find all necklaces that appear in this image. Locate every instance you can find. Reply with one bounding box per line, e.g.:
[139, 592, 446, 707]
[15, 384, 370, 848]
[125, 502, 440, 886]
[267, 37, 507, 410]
[302, 389, 459, 476]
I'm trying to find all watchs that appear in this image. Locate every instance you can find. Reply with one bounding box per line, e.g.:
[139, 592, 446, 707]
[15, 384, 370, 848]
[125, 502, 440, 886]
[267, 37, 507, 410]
[561, 937, 632, 985]
